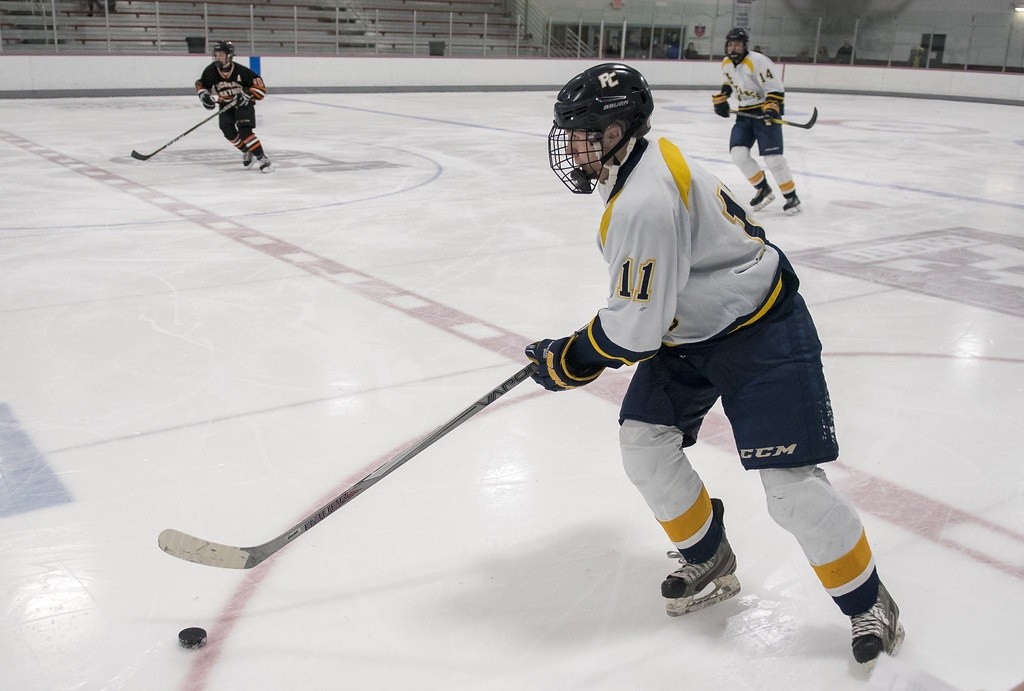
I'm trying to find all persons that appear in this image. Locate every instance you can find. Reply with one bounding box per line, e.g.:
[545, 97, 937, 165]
[524, 62, 905, 674]
[684, 43, 698, 59]
[606, 36, 620, 58]
[836, 39, 856, 64]
[711, 28, 803, 215]
[86, 0, 117, 17]
[796, 47, 814, 63]
[194, 40, 274, 173]
[752, 45, 763, 54]
[664, 32, 686, 59]
[816, 46, 832, 63]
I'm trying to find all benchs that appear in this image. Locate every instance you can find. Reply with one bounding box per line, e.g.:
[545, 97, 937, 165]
[0, 0, 554, 57]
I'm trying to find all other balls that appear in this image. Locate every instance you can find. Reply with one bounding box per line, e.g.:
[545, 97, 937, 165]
[178, 626, 208, 650]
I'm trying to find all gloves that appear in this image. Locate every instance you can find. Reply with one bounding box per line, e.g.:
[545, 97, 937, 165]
[524, 330, 604, 391]
[712, 95, 731, 118]
[762, 107, 781, 127]
[198, 92, 216, 110]
[235, 92, 254, 108]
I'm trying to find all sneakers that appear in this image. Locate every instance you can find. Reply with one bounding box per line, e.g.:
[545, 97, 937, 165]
[257, 154, 274, 173]
[850, 579, 905, 676]
[661, 496, 741, 617]
[783, 193, 801, 216]
[242, 151, 255, 169]
[750, 171, 776, 211]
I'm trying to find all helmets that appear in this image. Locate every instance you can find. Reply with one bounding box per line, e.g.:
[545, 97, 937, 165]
[724, 27, 749, 66]
[547, 62, 654, 194]
[212, 39, 235, 68]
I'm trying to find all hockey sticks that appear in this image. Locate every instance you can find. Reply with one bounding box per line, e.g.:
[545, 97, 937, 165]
[131, 96, 245, 162]
[729, 107, 819, 130]
[158, 319, 592, 569]
